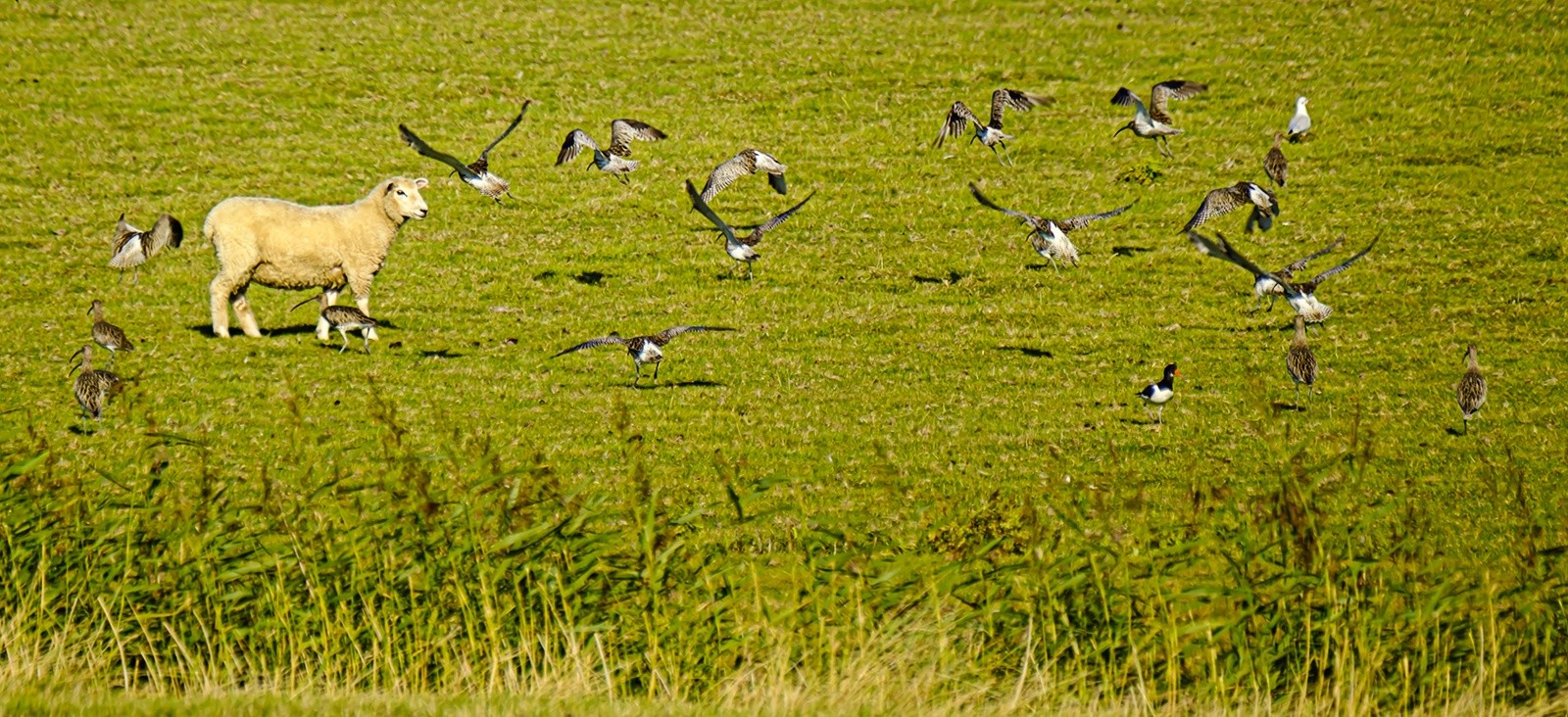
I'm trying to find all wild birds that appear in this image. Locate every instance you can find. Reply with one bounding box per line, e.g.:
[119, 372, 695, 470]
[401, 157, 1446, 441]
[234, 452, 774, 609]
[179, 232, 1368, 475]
[1111, 80, 1209, 162]
[688, 148, 790, 213]
[1285, 316, 1317, 414]
[1457, 344, 1489, 436]
[970, 180, 1142, 273]
[68, 345, 124, 424]
[1287, 96, 1313, 144]
[685, 179, 826, 282]
[549, 325, 736, 392]
[107, 212, 184, 283]
[1197, 229, 1390, 331]
[87, 299, 135, 364]
[1176, 180, 1280, 236]
[291, 295, 386, 357]
[556, 117, 670, 187]
[1262, 132, 1292, 188]
[1135, 363, 1184, 428]
[398, 97, 533, 209]
[930, 86, 1058, 170]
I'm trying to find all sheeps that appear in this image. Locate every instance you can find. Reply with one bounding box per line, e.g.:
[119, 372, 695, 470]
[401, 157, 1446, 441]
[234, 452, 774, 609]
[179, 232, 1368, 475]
[196, 172, 430, 343]
